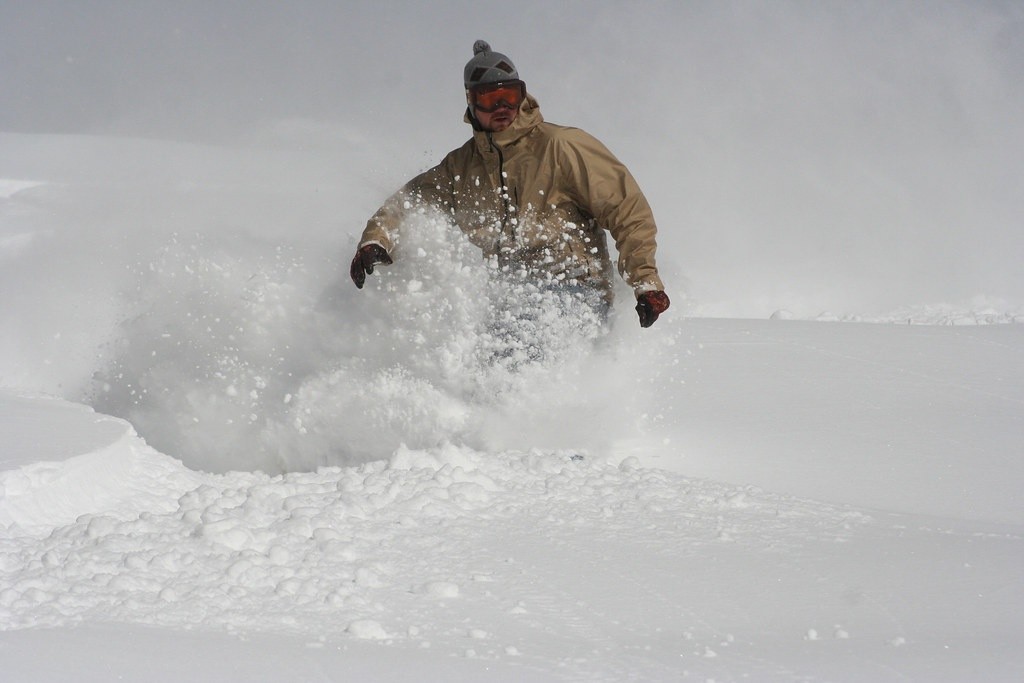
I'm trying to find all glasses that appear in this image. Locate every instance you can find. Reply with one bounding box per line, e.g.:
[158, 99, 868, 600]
[465, 80, 527, 114]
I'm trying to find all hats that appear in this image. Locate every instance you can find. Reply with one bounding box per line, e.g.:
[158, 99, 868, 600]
[463, 40, 521, 119]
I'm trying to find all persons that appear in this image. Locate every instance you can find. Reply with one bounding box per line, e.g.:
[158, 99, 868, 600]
[350, 40, 670, 367]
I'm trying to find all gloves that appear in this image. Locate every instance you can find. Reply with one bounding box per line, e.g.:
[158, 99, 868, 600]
[635, 289, 671, 328]
[349, 244, 393, 289]
